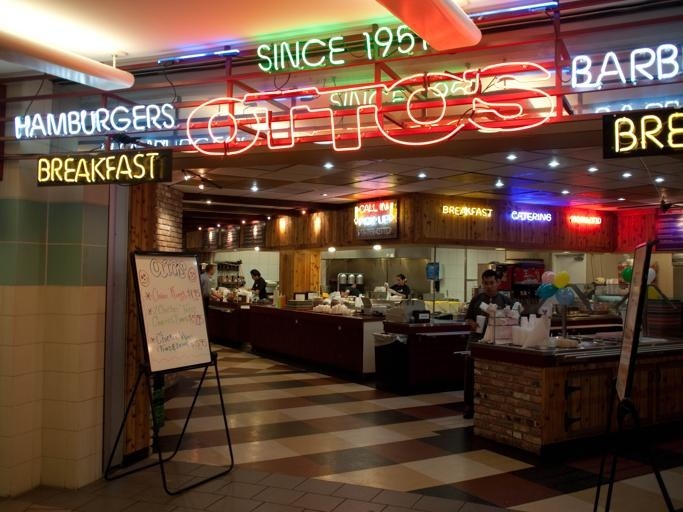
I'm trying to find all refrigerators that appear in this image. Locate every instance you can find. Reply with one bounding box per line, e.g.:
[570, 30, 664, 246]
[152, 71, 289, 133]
[477, 261, 545, 308]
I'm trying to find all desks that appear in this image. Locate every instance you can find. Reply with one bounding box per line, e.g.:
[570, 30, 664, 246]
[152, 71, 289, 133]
[472, 337, 683, 453]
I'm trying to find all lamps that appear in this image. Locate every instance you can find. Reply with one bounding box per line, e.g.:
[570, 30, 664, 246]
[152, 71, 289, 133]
[0, 31, 135, 92]
[373, 0, 481, 54]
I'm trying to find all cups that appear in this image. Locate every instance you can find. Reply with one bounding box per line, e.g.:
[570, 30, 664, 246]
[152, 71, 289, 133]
[476, 315, 486, 334]
[511, 314, 536, 344]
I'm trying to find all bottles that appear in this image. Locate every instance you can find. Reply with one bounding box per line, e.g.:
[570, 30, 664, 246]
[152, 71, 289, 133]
[232, 288, 238, 303]
[273, 288, 286, 308]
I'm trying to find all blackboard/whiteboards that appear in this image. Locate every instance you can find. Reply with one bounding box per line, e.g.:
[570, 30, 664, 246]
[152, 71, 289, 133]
[615, 241, 651, 404]
[132, 250, 213, 376]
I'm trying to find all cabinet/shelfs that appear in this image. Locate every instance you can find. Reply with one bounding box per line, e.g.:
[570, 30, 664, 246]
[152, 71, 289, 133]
[218, 260, 245, 290]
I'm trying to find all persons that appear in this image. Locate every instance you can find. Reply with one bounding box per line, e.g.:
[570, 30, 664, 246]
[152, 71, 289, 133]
[460, 269, 512, 420]
[247, 268, 268, 300]
[200, 264, 215, 309]
[210, 290, 222, 300]
[388, 274, 410, 299]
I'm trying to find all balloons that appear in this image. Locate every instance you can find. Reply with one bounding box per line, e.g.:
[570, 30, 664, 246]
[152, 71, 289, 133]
[534, 269, 574, 306]
[647, 268, 656, 285]
[622, 267, 632, 282]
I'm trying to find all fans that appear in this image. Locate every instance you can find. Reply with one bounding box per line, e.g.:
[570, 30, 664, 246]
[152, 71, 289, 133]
[617, 188, 683, 211]
